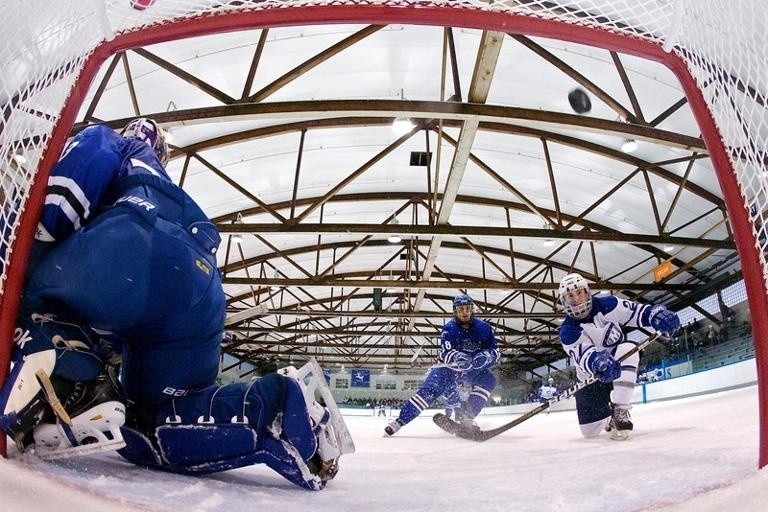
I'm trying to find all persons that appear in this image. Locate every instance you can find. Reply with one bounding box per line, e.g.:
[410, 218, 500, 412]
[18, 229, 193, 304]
[553, 273, 678, 437]
[519, 303, 753, 404]
[4, 117, 339, 495]
[344, 390, 499, 420]
[379, 296, 495, 443]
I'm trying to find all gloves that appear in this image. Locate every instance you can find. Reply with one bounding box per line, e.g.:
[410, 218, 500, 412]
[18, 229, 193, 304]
[472, 349, 492, 369]
[591, 349, 621, 383]
[453, 352, 473, 370]
[648, 304, 680, 340]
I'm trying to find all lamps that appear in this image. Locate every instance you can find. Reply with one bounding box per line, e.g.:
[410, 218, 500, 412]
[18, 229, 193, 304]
[543, 223, 554, 247]
[391, 89, 413, 138]
[388, 212, 401, 244]
[617, 114, 638, 153]
[231, 213, 242, 242]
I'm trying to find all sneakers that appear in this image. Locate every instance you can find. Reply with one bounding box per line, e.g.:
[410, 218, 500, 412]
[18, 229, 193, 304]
[385, 418, 403, 435]
[605, 403, 633, 432]
[16, 364, 126, 452]
[460, 414, 480, 431]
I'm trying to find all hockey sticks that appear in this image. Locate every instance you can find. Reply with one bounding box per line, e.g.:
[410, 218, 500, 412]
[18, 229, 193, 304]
[433, 330, 662, 441]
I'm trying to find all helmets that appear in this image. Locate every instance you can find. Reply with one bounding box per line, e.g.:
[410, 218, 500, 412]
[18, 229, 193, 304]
[453, 295, 474, 324]
[559, 273, 593, 320]
[120, 117, 170, 169]
